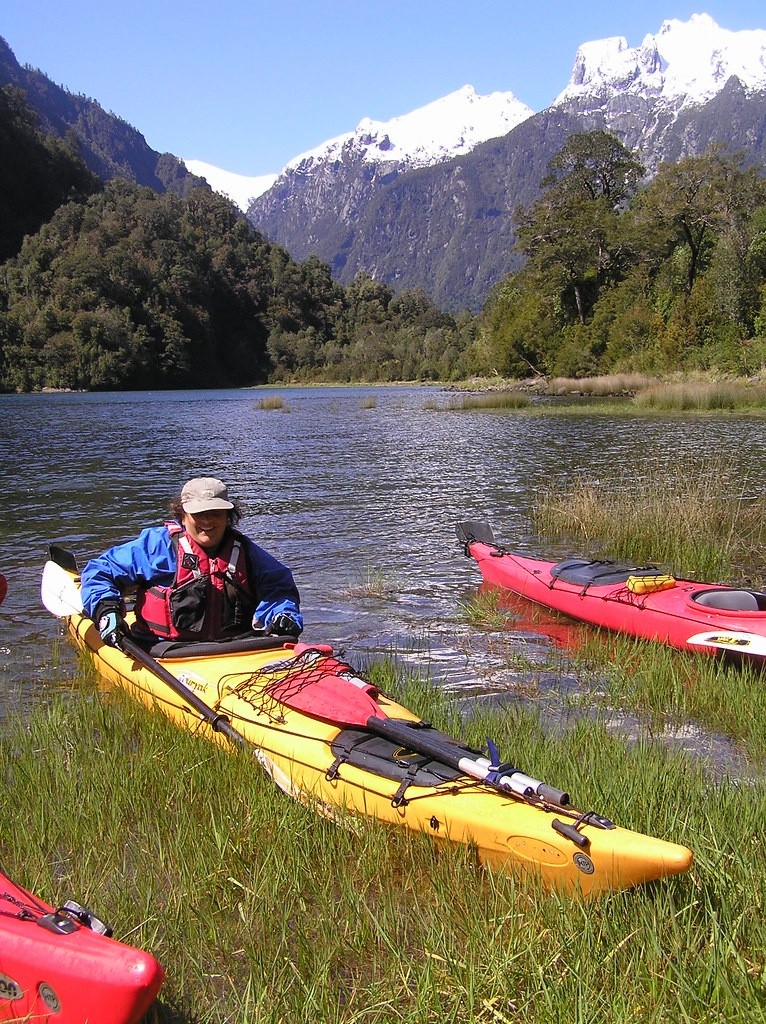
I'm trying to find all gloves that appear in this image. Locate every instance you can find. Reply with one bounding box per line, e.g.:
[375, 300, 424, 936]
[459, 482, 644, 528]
[265, 613, 298, 636]
[99, 609, 130, 652]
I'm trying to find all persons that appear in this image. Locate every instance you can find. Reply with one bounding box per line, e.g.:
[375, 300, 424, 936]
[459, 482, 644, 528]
[80, 478, 304, 652]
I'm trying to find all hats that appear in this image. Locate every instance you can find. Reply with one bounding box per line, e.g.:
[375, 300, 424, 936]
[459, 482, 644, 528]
[180, 477, 234, 514]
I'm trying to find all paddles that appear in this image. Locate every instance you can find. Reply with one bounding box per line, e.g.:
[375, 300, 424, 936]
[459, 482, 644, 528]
[39, 560, 365, 841]
[685, 630, 766, 656]
[262, 669, 571, 807]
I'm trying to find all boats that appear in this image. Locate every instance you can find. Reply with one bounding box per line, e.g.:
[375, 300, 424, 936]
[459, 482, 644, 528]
[451, 518, 766, 668]
[0, 868, 167, 1024]
[35, 544, 691, 894]
